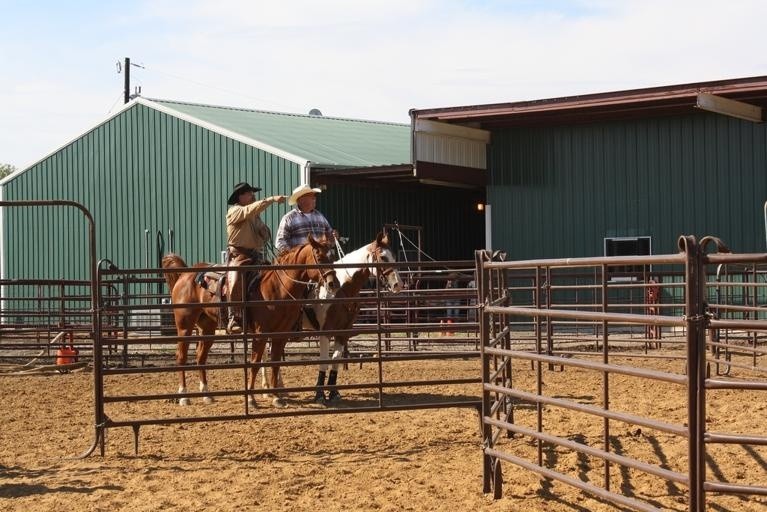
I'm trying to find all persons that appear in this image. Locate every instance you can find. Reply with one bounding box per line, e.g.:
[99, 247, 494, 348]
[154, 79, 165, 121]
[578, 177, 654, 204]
[275, 184, 339, 264]
[225, 182, 289, 333]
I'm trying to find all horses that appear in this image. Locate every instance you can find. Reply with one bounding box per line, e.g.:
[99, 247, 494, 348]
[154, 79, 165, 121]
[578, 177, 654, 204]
[161, 230, 340, 409]
[261, 231, 405, 401]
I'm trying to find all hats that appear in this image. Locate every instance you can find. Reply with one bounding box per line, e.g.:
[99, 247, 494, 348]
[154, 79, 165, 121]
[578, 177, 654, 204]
[227, 182, 261, 205]
[288, 183, 321, 206]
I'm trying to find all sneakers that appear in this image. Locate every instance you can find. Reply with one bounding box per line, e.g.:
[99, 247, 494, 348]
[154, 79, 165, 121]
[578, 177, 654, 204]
[226, 321, 243, 334]
